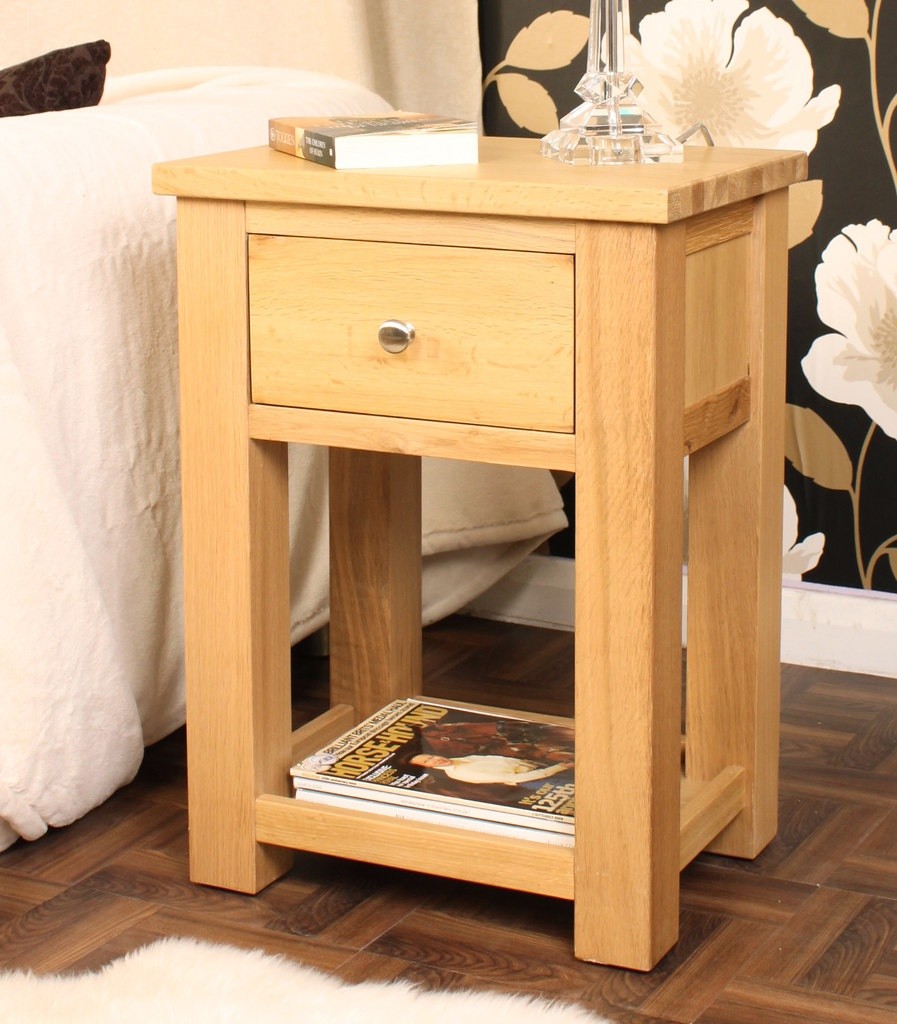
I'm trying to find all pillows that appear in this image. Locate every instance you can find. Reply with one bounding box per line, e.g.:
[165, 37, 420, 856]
[2, 35, 113, 116]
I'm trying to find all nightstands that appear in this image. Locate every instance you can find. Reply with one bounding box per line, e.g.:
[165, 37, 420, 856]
[151, 113, 813, 973]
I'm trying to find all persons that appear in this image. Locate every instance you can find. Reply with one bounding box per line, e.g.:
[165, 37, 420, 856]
[397, 750, 574, 790]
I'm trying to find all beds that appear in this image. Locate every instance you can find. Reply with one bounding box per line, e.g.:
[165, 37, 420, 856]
[4, 68, 566, 861]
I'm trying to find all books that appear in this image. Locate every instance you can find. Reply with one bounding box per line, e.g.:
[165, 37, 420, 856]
[267, 108, 478, 171]
[288, 699, 576, 847]
